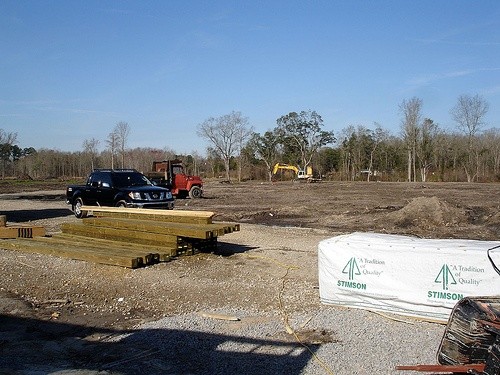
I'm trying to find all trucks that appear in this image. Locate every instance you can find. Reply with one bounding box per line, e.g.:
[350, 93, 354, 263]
[139, 158, 204, 200]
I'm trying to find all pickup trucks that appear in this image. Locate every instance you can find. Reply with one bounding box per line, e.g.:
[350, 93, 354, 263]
[66, 167, 175, 220]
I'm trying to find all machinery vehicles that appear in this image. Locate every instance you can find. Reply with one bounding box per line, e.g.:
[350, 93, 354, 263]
[273, 163, 309, 180]
